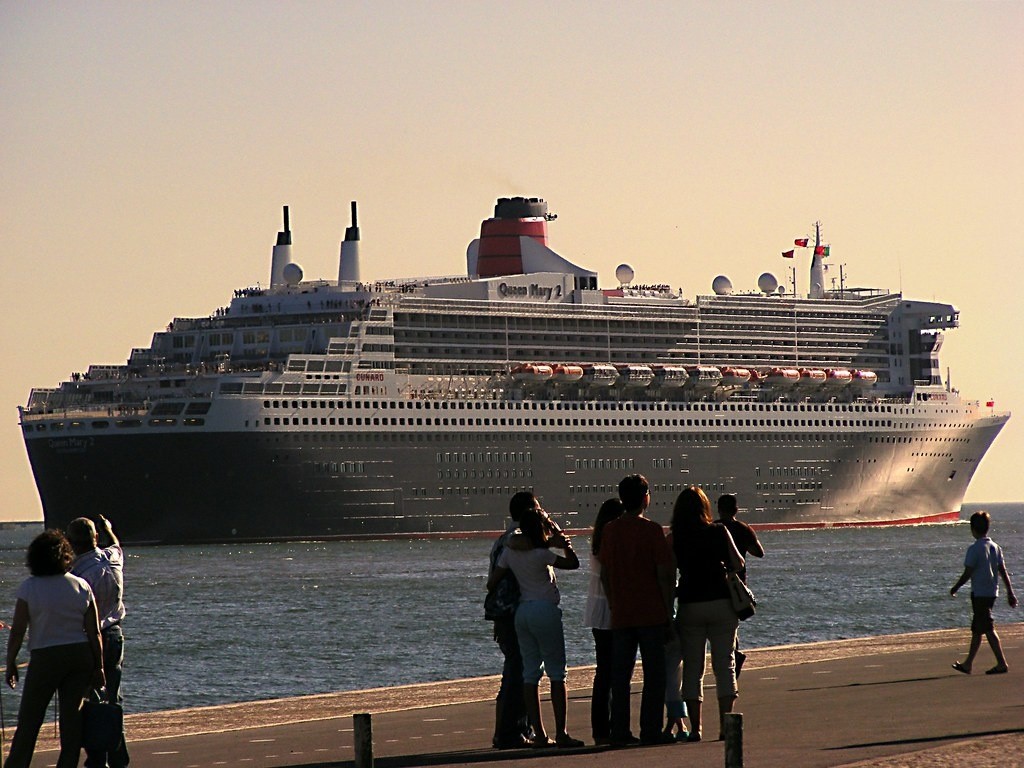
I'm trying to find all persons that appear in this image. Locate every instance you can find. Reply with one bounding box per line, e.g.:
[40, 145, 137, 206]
[597, 473, 673, 743]
[661, 631, 690, 739]
[665, 485, 744, 740]
[66, 512, 130, 768]
[582, 498, 624, 746]
[711, 494, 764, 680]
[483, 492, 585, 748]
[0, 529, 106, 768]
[949, 510, 1019, 675]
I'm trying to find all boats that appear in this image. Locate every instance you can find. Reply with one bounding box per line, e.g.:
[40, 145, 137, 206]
[851, 366, 878, 387]
[612, 360, 657, 389]
[762, 366, 801, 387]
[550, 359, 584, 382]
[822, 365, 853, 385]
[647, 361, 693, 390]
[511, 361, 554, 382]
[718, 365, 752, 385]
[683, 362, 725, 388]
[580, 360, 621, 386]
[797, 365, 827, 385]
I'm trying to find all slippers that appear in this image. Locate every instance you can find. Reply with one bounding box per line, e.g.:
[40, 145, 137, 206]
[985, 666, 1009, 675]
[952, 661, 971, 674]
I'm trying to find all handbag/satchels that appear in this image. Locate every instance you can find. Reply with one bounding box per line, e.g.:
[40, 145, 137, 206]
[718, 522, 757, 622]
[80, 684, 123, 751]
[484, 566, 521, 620]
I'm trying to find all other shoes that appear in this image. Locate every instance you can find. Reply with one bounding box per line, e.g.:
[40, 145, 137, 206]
[609, 735, 639, 746]
[492, 736, 533, 750]
[682, 733, 701, 742]
[532, 734, 556, 747]
[663, 731, 675, 743]
[593, 736, 609, 745]
[675, 731, 689, 741]
[556, 734, 584, 748]
[719, 733, 726, 742]
[640, 733, 676, 745]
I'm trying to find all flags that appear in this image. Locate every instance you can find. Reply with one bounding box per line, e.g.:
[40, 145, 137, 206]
[782, 250, 794, 258]
[815, 245, 830, 256]
[794, 238, 808, 247]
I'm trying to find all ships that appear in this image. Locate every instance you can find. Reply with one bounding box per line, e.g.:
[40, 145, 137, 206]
[16, 197, 1012, 556]
